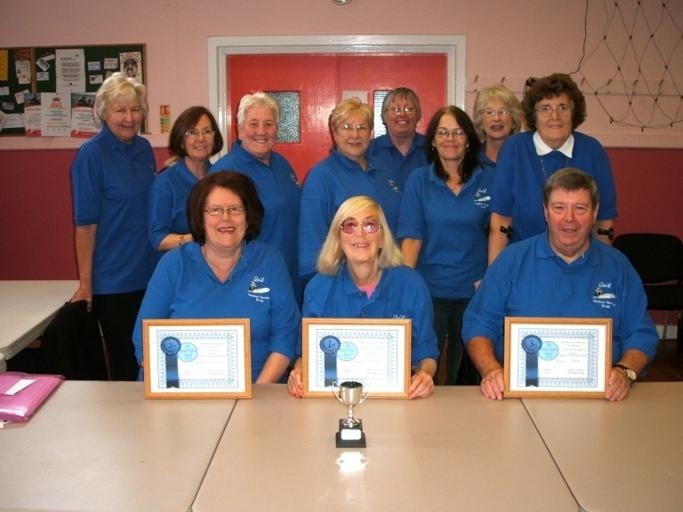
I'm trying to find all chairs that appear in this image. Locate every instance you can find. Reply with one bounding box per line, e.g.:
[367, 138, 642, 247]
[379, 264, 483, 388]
[613, 234, 683, 371]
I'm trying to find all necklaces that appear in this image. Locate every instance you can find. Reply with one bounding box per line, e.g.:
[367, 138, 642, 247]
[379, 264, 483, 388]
[540, 155, 570, 183]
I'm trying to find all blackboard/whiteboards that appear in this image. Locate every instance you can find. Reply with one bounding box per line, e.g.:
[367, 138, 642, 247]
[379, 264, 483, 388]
[0, 43, 149, 137]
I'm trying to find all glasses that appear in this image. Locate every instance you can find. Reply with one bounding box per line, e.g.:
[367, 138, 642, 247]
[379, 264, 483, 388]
[185, 129, 215, 137]
[537, 105, 570, 117]
[387, 106, 418, 115]
[339, 221, 383, 233]
[481, 112, 514, 121]
[432, 128, 470, 147]
[336, 124, 371, 136]
[203, 207, 246, 215]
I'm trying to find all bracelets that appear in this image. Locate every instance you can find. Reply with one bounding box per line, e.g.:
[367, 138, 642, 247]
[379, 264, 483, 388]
[178, 235, 184, 246]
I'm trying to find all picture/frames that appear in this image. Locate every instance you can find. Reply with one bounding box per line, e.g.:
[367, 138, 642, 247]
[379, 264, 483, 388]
[504, 316, 613, 399]
[142, 319, 252, 399]
[301, 318, 412, 400]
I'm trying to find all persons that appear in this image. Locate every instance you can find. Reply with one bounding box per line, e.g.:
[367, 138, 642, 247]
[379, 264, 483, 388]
[285, 193, 442, 401]
[395, 105, 495, 386]
[472, 72, 618, 293]
[144, 106, 224, 252]
[297, 96, 402, 283]
[207, 90, 301, 310]
[371, 85, 426, 194]
[65, 70, 156, 380]
[472, 83, 524, 165]
[458, 165, 659, 403]
[130, 169, 301, 384]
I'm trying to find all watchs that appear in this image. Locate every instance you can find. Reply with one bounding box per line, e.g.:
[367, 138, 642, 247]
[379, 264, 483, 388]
[612, 364, 638, 384]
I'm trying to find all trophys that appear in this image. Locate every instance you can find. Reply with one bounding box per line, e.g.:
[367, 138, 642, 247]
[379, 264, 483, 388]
[329, 378, 369, 449]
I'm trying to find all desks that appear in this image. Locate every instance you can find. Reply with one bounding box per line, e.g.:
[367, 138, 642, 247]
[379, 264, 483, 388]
[190, 384, 584, 512]
[0, 380, 237, 512]
[0, 280, 81, 372]
[520, 381, 683, 512]
